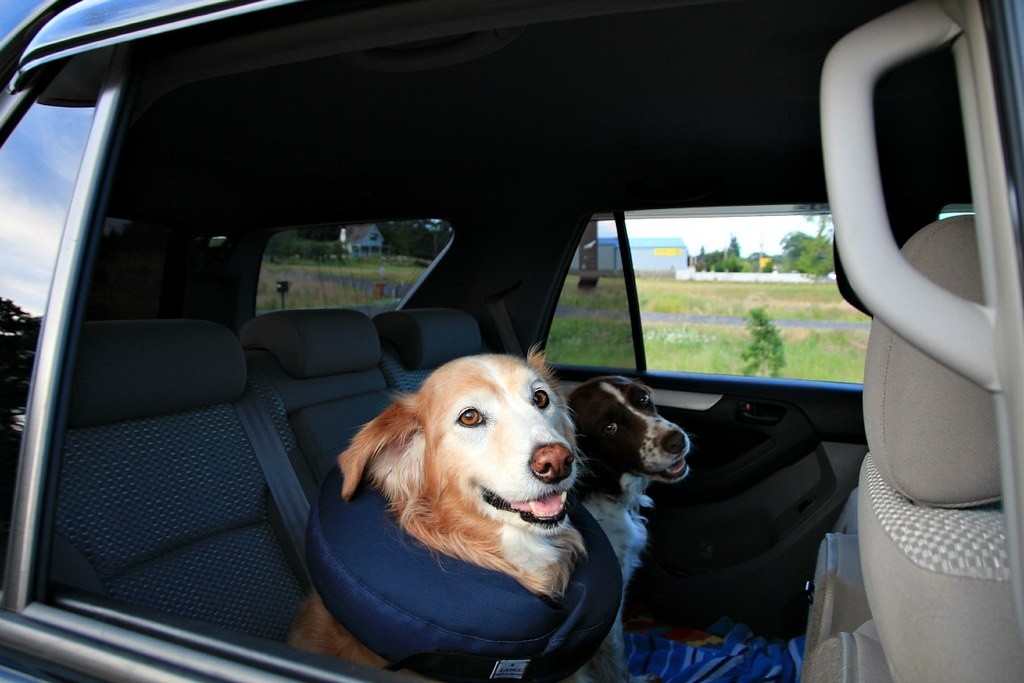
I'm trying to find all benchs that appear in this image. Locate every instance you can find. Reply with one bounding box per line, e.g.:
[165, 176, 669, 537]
[50, 303, 487, 644]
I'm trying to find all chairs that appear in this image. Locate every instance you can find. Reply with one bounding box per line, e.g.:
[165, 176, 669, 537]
[856, 212, 1024, 683]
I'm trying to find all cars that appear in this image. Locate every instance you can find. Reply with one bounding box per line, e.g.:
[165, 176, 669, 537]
[0, 0, 1024, 683]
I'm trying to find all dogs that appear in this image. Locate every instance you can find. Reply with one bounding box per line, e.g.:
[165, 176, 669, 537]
[567, 376, 691, 683]
[287, 338, 597, 683]
